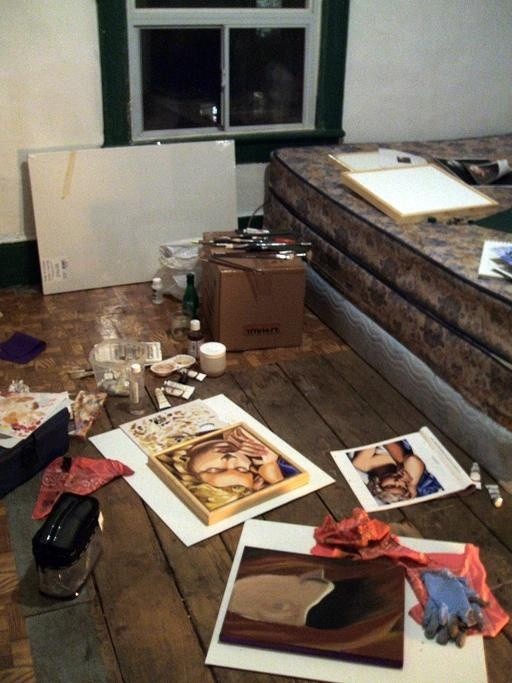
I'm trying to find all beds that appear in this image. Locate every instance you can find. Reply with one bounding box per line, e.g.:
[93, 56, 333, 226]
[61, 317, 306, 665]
[264, 131, 512, 486]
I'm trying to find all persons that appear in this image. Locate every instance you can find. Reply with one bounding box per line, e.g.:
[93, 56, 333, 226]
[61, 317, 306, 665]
[352, 439, 445, 506]
[160, 425, 301, 510]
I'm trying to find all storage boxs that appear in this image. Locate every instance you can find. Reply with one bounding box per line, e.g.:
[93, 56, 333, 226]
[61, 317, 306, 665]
[202, 231, 304, 351]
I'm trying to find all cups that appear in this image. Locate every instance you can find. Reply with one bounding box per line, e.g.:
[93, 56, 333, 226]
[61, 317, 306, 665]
[198, 341, 226, 377]
[170, 311, 191, 341]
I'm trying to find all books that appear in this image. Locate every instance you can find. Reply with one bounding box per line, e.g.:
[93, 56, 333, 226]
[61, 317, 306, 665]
[478, 239, 512, 282]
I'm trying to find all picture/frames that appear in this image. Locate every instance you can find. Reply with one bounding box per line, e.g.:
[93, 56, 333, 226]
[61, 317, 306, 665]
[327, 141, 499, 225]
[147, 421, 310, 525]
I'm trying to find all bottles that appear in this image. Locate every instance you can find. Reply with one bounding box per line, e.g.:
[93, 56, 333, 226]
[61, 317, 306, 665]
[181, 273, 198, 316]
[188, 319, 202, 360]
[127, 364, 147, 416]
[150, 278, 165, 305]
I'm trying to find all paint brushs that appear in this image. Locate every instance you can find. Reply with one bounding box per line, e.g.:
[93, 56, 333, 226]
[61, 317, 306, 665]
[66, 364, 152, 381]
[191, 234, 313, 264]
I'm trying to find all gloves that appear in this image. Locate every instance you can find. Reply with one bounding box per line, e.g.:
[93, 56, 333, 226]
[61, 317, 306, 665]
[423, 575, 484, 648]
[421, 567, 488, 638]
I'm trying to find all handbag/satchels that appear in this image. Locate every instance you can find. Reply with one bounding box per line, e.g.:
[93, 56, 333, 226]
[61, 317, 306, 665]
[31, 491, 105, 601]
